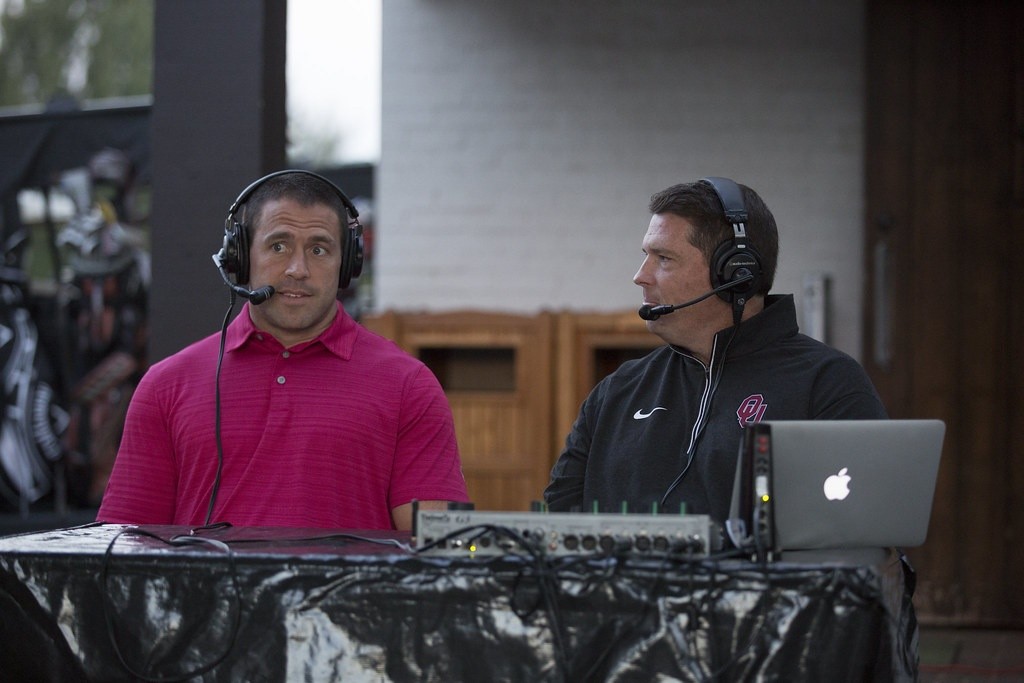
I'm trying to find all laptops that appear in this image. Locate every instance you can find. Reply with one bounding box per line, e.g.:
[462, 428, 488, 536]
[727, 418, 945, 548]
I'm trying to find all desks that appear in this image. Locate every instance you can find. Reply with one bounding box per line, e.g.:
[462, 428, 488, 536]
[0, 523, 924, 683]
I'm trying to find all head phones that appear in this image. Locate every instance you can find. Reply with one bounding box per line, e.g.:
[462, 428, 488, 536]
[698, 175, 770, 303]
[223, 168, 364, 290]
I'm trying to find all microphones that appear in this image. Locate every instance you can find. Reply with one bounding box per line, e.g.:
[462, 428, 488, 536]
[639, 268, 754, 321]
[212, 248, 276, 305]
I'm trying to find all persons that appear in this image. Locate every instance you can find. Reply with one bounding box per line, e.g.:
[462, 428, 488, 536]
[53, 170, 472, 531]
[544, 176, 889, 546]
